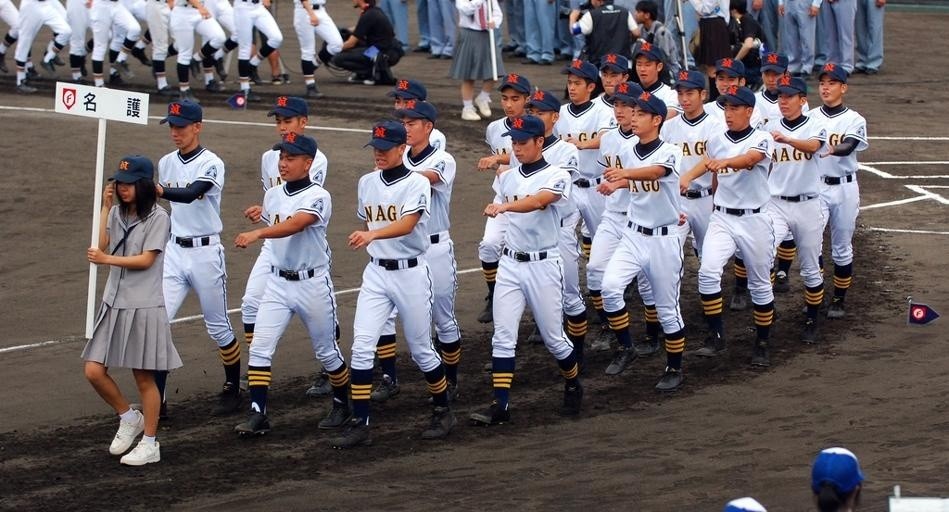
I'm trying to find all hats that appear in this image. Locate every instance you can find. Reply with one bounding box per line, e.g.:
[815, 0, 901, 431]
[499, 73, 530, 94]
[273, 132, 317, 157]
[268, 97, 307, 117]
[395, 101, 437, 122]
[387, 79, 426, 99]
[160, 99, 203, 126]
[502, 115, 544, 140]
[107, 155, 153, 183]
[524, 89, 561, 111]
[812, 447, 863, 493]
[561, 43, 667, 118]
[364, 120, 406, 150]
[671, 53, 847, 106]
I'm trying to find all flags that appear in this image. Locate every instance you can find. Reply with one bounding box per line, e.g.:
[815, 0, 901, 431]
[907, 302, 942, 325]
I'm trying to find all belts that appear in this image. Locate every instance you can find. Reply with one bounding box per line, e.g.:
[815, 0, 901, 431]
[782, 195, 815, 202]
[370, 257, 418, 269]
[169, 233, 209, 247]
[823, 174, 853, 186]
[430, 233, 438, 242]
[271, 266, 313, 281]
[576, 178, 599, 187]
[716, 205, 760, 216]
[502, 247, 546, 261]
[682, 188, 713, 198]
[628, 221, 667, 235]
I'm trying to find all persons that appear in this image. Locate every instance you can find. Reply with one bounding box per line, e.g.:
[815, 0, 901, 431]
[810, 446, 866, 511]
[725, 497, 767, 512]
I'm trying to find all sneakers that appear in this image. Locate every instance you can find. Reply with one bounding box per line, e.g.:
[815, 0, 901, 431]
[477, 293, 492, 323]
[0, 45, 291, 103]
[109, 410, 144, 456]
[558, 382, 583, 414]
[211, 380, 242, 416]
[592, 322, 688, 393]
[234, 408, 273, 438]
[470, 403, 511, 427]
[462, 107, 480, 121]
[730, 270, 845, 343]
[475, 97, 491, 117]
[503, 44, 550, 65]
[751, 338, 771, 366]
[414, 45, 450, 60]
[854, 66, 876, 74]
[695, 332, 728, 357]
[120, 440, 161, 466]
[306, 366, 400, 450]
[307, 84, 325, 98]
[422, 407, 457, 438]
[428, 381, 460, 404]
